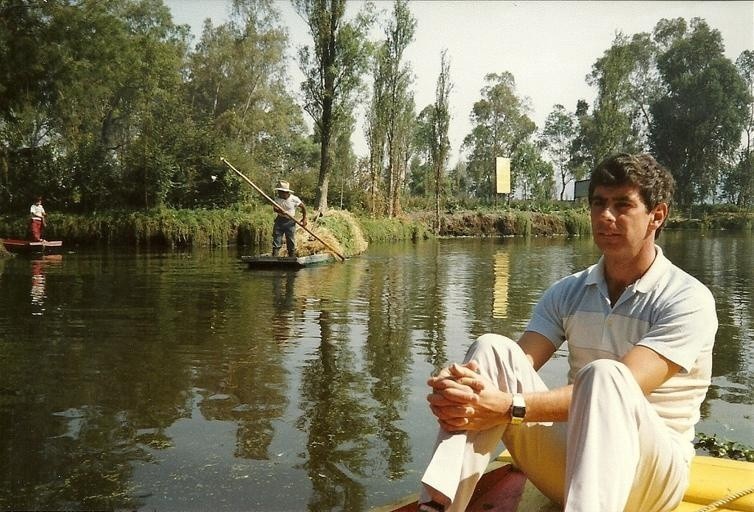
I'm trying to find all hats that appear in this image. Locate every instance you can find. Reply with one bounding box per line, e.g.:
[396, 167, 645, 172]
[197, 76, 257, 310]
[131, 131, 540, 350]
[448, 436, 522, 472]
[273, 181, 295, 194]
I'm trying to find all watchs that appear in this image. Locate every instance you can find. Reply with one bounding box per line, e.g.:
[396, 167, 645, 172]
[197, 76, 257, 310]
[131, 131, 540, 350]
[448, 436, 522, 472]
[509, 390, 527, 425]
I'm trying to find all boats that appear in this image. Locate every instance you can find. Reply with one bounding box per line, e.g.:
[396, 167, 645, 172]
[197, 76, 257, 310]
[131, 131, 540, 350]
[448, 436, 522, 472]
[359, 441, 754, 512]
[240, 249, 334, 267]
[10, 255, 62, 263]
[3, 235, 66, 259]
[247, 266, 330, 277]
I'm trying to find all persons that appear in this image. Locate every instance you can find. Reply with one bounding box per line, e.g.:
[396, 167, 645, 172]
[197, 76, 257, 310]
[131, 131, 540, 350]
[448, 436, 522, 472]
[272, 180, 307, 256]
[29, 195, 47, 242]
[413, 152, 719, 512]
